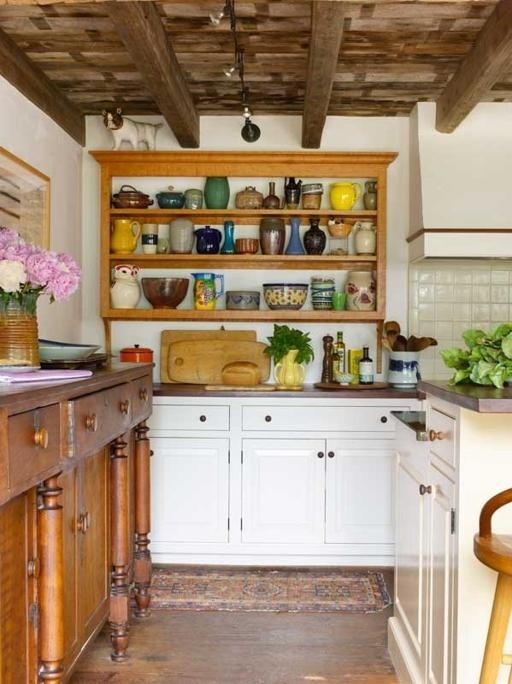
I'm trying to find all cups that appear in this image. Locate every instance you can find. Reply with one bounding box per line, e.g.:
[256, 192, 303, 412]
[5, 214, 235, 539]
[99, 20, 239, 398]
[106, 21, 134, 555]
[191, 273, 225, 311]
[158, 223, 170, 254]
[259, 218, 285, 255]
[332, 291, 347, 310]
[141, 223, 158, 254]
[387, 351, 420, 387]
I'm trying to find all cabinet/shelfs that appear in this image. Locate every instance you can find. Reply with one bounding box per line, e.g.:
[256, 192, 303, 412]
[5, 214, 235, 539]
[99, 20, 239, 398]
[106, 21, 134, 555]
[237, 396, 423, 568]
[88, 149, 401, 375]
[2, 362, 158, 684]
[417, 379, 511, 683]
[387, 410, 426, 683]
[147, 396, 236, 565]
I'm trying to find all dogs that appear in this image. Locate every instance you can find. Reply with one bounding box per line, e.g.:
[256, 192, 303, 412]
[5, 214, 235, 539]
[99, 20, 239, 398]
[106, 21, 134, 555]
[101, 106, 163, 150]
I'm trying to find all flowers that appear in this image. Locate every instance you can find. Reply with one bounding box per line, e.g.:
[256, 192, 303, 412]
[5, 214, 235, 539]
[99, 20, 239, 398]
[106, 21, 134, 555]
[1, 228, 82, 302]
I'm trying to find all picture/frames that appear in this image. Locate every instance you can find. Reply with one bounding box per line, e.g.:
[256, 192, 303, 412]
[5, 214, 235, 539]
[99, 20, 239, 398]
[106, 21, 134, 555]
[0, 145, 52, 252]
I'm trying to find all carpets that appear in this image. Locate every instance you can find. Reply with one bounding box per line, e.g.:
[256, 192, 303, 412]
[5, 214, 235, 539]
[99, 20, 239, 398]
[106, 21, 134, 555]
[127, 566, 393, 612]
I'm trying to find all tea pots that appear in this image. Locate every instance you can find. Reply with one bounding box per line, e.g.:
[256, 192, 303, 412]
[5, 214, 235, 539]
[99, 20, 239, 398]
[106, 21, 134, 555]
[364, 181, 378, 210]
[283, 176, 303, 210]
[111, 218, 141, 254]
[235, 185, 264, 209]
[111, 185, 154, 208]
[155, 184, 187, 209]
[194, 225, 221, 253]
[329, 181, 362, 210]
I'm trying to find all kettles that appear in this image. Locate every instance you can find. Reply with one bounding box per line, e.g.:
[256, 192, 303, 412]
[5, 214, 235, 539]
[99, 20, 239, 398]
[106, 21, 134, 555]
[110, 272, 140, 308]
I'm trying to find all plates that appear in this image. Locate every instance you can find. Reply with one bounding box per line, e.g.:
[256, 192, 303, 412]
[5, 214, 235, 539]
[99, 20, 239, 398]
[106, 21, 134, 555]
[0, 365, 42, 375]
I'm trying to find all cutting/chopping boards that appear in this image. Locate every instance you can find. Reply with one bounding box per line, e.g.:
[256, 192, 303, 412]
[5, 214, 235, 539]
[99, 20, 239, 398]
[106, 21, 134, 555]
[159, 328, 256, 385]
[168, 339, 272, 386]
[205, 383, 304, 390]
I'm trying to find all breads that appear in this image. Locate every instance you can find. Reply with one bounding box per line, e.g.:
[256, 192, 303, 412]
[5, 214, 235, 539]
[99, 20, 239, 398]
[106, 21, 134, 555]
[221, 360, 264, 386]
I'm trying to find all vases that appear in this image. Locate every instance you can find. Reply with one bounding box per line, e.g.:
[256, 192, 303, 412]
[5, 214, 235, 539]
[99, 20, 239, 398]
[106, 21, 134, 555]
[363, 180, 376, 209]
[0, 287, 43, 373]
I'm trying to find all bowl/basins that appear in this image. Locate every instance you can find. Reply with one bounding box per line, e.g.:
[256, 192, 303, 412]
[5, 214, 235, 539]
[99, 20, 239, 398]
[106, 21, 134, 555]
[328, 223, 354, 237]
[226, 291, 260, 309]
[333, 373, 355, 387]
[36, 338, 102, 359]
[311, 276, 335, 310]
[141, 277, 189, 310]
[262, 281, 309, 310]
[235, 238, 258, 255]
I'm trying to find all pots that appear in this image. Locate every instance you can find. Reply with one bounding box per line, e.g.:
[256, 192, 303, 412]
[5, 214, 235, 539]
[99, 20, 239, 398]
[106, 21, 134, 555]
[120, 343, 153, 362]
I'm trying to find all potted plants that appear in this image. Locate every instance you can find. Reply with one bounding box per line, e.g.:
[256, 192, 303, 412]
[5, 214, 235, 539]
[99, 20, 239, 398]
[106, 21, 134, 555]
[263, 322, 316, 390]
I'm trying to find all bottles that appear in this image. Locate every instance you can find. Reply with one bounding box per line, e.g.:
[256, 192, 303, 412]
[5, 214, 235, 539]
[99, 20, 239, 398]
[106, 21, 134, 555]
[320, 333, 333, 381]
[357, 344, 375, 384]
[330, 236, 349, 255]
[345, 269, 376, 310]
[331, 344, 340, 373]
[205, 176, 230, 208]
[337, 330, 346, 372]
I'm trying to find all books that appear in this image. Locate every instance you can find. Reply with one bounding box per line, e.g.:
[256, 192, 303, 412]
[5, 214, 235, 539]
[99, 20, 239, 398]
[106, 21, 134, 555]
[1, 368, 96, 386]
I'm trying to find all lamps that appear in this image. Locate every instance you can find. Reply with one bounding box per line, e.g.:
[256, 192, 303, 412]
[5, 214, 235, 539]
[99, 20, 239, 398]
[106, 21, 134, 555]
[209, 0, 262, 142]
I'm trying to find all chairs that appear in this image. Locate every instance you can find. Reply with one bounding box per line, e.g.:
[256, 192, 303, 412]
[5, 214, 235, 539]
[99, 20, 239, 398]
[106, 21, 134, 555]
[473, 488, 511, 682]
